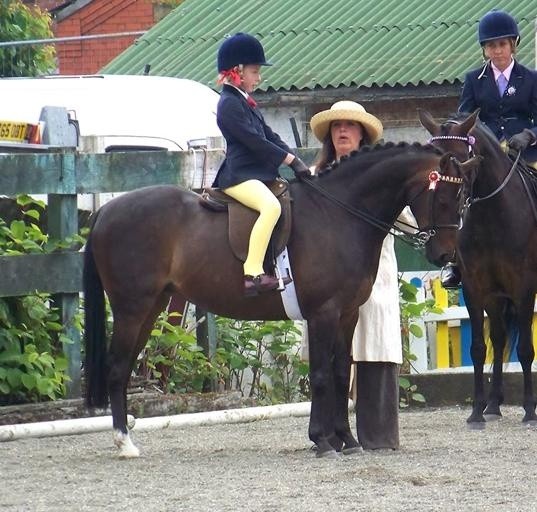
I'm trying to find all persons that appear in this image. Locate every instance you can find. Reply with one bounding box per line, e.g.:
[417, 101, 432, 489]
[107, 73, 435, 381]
[440, 10, 536, 287]
[213, 30, 310, 299]
[305, 98, 420, 450]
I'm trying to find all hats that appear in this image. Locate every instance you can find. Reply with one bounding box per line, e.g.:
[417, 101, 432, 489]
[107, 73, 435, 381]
[310, 101, 383, 144]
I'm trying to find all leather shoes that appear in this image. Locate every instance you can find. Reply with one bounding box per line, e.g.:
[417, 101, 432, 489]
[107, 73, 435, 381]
[442, 264, 462, 287]
[240, 274, 291, 299]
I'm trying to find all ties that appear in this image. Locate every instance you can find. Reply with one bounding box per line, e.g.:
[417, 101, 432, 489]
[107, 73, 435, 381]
[498, 74, 507, 97]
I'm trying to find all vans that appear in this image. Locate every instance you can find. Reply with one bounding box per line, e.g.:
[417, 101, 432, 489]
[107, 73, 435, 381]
[0, 74, 227, 318]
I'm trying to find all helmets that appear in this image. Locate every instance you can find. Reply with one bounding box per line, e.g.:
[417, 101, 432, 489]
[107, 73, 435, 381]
[218, 33, 273, 73]
[478, 10, 520, 46]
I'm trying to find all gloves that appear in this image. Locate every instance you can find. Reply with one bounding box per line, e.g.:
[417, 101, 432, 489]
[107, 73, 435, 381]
[288, 155, 311, 177]
[509, 128, 535, 152]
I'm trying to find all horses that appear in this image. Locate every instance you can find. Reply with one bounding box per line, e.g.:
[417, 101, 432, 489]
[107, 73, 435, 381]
[415, 105, 537, 436]
[81, 138, 466, 462]
